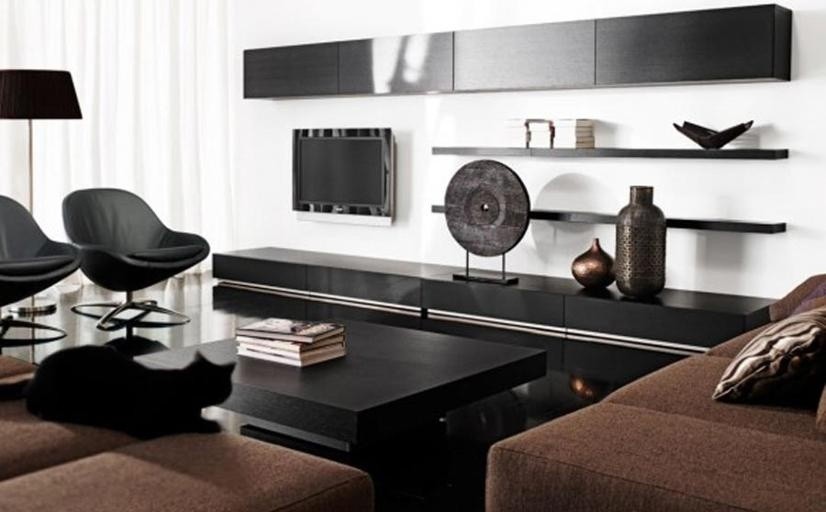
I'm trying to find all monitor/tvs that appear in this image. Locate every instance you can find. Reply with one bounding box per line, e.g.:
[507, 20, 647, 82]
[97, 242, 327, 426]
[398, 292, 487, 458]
[292, 127, 394, 227]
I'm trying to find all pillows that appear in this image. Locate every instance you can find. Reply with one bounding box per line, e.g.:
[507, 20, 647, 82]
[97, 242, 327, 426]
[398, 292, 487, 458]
[710, 305, 825, 404]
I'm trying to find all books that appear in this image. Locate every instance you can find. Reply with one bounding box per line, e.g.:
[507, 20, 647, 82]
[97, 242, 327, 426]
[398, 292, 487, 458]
[237, 342, 344, 361]
[234, 315, 345, 344]
[521, 116, 596, 150]
[235, 334, 344, 352]
[235, 346, 348, 369]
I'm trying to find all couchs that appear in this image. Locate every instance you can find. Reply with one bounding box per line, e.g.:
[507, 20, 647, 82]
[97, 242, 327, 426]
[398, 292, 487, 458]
[0, 353, 375, 512]
[488, 274, 826, 510]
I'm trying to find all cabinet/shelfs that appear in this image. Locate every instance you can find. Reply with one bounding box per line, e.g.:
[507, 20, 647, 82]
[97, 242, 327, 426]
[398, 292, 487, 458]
[430, 136, 792, 236]
[242, 3, 794, 99]
[209, 244, 787, 389]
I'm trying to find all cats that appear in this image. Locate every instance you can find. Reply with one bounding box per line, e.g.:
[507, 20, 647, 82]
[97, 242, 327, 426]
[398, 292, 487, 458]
[0, 344, 238, 442]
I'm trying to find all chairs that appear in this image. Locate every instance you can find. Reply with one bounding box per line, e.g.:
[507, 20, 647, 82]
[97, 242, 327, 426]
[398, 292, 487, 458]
[0, 185, 210, 347]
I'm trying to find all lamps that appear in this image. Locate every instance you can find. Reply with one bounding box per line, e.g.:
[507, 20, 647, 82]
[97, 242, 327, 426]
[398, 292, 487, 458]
[0, 66, 86, 316]
